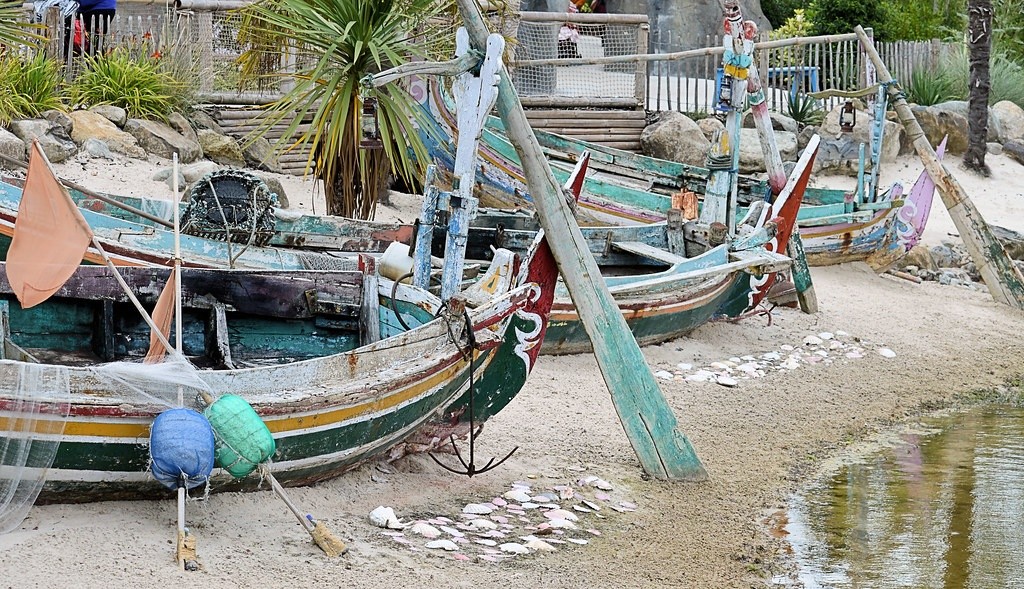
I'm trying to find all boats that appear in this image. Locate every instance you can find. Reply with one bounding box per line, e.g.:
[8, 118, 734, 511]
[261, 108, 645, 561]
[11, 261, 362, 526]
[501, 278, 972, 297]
[1, 18, 949, 507]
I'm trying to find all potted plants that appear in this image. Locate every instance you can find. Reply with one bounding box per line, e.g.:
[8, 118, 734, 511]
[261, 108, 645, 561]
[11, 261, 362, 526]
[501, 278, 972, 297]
[234, 1, 470, 222]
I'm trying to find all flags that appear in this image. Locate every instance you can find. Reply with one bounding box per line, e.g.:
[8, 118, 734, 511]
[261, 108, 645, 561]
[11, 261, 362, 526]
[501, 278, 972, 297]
[6, 137, 94, 309]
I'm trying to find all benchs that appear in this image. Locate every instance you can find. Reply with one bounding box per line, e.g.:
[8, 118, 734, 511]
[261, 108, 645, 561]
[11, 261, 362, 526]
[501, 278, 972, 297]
[606, 240, 688, 265]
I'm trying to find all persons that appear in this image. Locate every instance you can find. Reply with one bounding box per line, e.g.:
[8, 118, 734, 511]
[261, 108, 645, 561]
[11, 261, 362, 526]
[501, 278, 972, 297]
[73, 0, 116, 72]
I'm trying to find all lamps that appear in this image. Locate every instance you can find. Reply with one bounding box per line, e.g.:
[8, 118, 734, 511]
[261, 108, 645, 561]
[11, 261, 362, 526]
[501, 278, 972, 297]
[840, 101, 856, 133]
